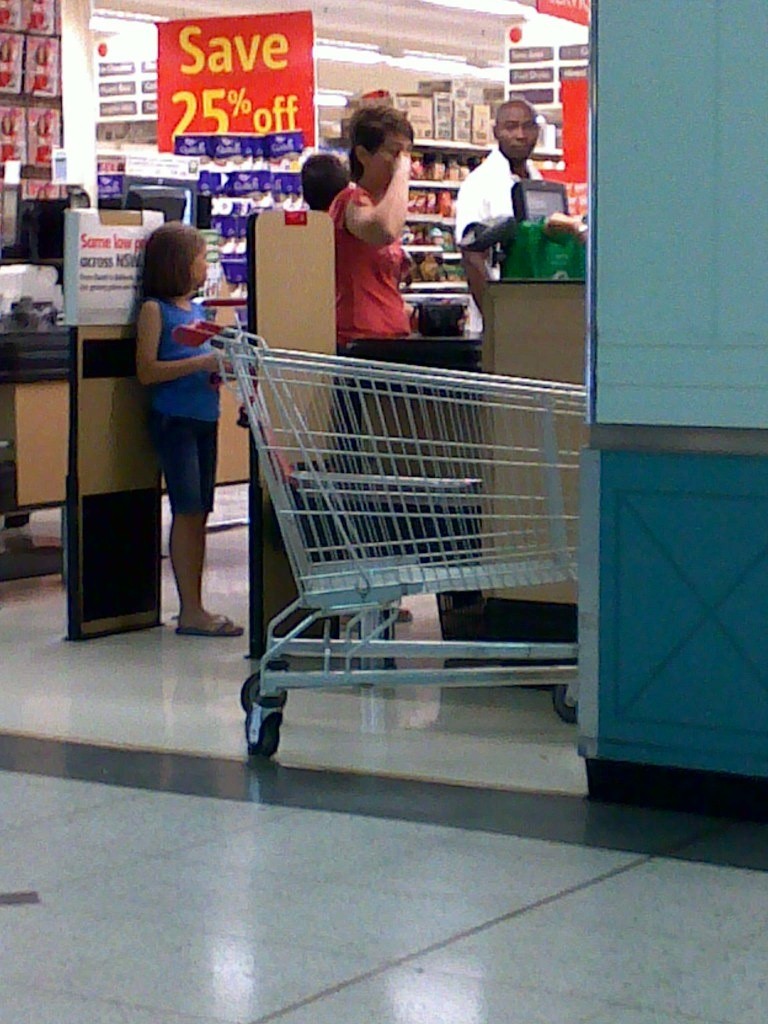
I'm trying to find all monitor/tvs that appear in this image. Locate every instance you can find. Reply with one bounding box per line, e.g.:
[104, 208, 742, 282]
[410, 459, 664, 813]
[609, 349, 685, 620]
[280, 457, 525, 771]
[512, 181, 569, 224]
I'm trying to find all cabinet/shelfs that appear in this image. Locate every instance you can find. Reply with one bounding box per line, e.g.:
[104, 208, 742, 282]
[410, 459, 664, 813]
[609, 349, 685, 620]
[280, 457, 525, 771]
[0, 0, 590, 335]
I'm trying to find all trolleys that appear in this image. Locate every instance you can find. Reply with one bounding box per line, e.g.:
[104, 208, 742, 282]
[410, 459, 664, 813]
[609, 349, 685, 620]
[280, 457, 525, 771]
[171, 319, 583, 777]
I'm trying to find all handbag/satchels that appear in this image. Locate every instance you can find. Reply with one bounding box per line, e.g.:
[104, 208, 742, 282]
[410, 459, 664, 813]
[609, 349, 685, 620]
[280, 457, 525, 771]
[500, 216, 585, 280]
[417, 297, 469, 336]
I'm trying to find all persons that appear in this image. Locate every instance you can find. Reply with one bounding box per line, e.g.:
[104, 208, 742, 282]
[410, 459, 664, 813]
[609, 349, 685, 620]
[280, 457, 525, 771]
[301, 105, 471, 623]
[134, 224, 245, 638]
[453, 99, 589, 339]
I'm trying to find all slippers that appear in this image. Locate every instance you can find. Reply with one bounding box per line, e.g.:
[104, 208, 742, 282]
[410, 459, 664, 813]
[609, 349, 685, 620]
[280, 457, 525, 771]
[175, 613, 243, 637]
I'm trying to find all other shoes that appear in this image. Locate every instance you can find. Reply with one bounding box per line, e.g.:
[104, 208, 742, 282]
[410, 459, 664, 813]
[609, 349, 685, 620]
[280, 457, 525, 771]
[337, 615, 359, 632]
[382, 606, 412, 621]
